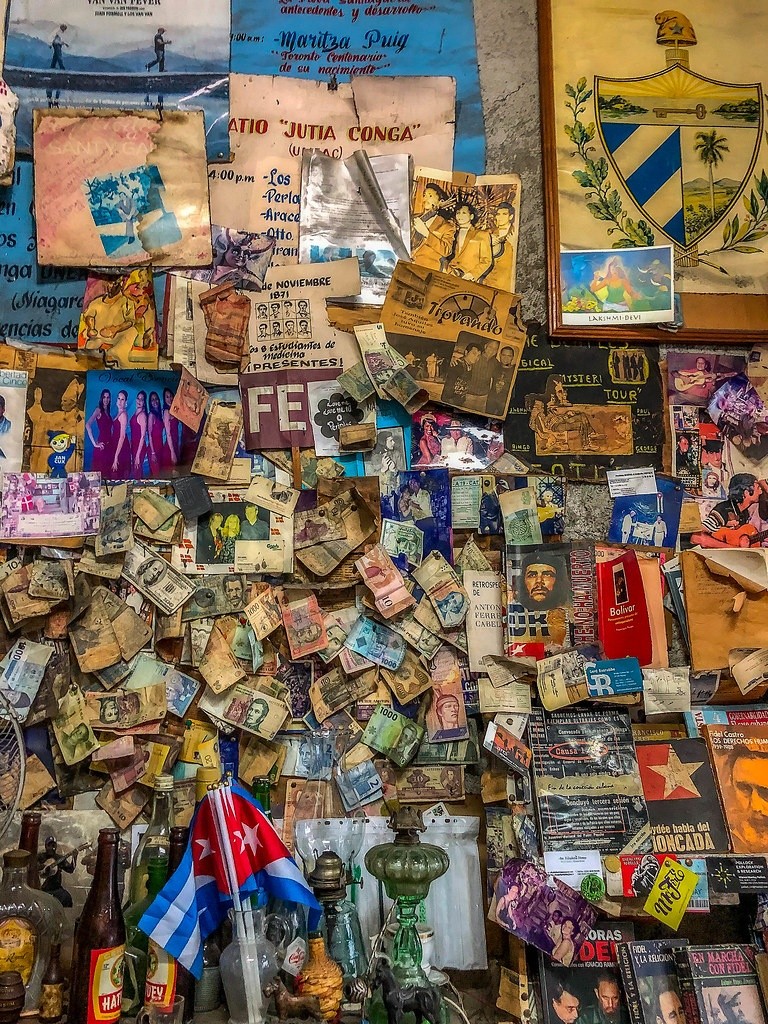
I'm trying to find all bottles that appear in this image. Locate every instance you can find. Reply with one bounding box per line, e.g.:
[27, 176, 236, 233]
[0, 766, 303, 1023]
[298, 931, 344, 1020]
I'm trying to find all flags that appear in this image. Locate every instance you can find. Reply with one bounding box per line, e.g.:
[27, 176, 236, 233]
[138, 777, 324, 982]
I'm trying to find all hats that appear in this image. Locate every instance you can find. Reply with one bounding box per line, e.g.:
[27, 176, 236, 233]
[497, 480, 509, 489]
[61, 378, 85, 400]
[521, 552, 566, 575]
[701, 434, 725, 453]
[445, 420, 464, 430]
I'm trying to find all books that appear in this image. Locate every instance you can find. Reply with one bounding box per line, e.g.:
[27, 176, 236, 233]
[543, 920, 767, 1024]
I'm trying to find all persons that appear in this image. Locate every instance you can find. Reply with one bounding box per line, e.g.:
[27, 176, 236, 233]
[441, 339, 517, 416]
[405, 351, 441, 378]
[27, 378, 85, 472]
[690, 473, 768, 548]
[416, 414, 502, 470]
[398, 478, 434, 557]
[116, 191, 143, 244]
[479, 479, 510, 533]
[574, 971, 631, 1024]
[496, 882, 578, 967]
[366, 431, 404, 495]
[553, 971, 583, 1024]
[673, 407, 726, 497]
[315, 248, 396, 278]
[411, 183, 515, 293]
[621, 511, 667, 546]
[86, 389, 180, 479]
[536, 490, 563, 535]
[669, 357, 718, 405]
[656, 986, 686, 1024]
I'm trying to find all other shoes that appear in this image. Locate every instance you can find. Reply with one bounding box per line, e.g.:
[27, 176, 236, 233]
[144, 65, 151, 72]
[159, 69, 168, 72]
